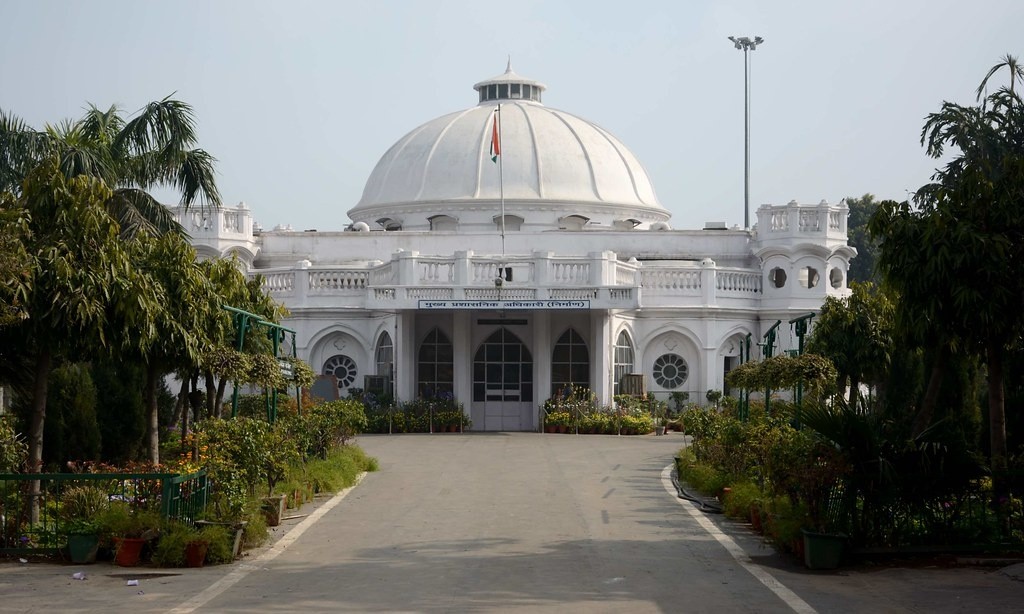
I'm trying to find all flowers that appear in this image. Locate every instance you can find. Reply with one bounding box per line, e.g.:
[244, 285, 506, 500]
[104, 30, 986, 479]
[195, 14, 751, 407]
[353, 395, 473, 434]
[545, 384, 653, 430]
[67, 432, 212, 509]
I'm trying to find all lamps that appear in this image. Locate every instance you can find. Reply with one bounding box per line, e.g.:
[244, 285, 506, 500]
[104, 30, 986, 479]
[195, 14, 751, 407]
[495, 277, 502, 286]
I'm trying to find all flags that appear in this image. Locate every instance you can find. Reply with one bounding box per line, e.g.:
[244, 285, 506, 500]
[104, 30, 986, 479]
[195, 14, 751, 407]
[489, 111, 500, 164]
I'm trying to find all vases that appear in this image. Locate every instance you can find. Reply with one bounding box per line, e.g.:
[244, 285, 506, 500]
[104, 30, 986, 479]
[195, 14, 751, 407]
[432, 424, 436, 432]
[610, 426, 617, 435]
[459, 425, 464, 432]
[589, 426, 597, 434]
[635, 430, 640, 435]
[621, 427, 627, 434]
[449, 424, 457, 432]
[628, 428, 636, 435]
[548, 424, 557, 432]
[655, 427, 666, 435]
[558, 424, 567, 433]
[439, 424, 447, 432]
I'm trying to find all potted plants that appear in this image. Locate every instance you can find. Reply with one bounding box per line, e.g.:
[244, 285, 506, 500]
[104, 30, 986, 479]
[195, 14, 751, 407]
[673, 431, 862, 573]
[57, 443, 377, 566]
[660, 417, 669, 434]
